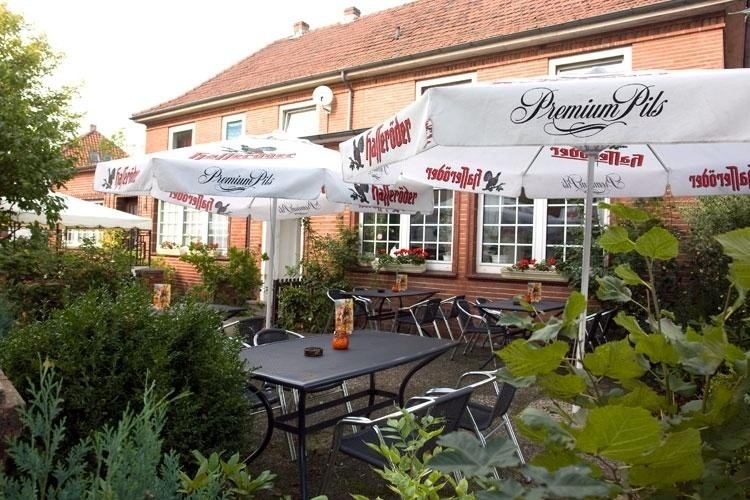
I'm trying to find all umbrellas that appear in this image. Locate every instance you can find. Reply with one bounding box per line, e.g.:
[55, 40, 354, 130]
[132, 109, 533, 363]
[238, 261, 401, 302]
[335, 61, 750, 418]
[91, 131, 439, 334]
[0, 191, 156, 241]
[395, 193, 602, 268]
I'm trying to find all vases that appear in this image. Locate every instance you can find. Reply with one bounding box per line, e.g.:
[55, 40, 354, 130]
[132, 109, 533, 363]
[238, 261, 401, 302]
[499, 267, 571, 283]
[378, 261, 427, 274]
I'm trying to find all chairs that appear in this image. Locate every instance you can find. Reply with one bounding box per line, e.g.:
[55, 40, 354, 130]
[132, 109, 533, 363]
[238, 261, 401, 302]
[318, 387, 474, 495]
[217, 315, 265, 346]
[453, 296, 618, 370]
[221, 338, 297, 461]
[422, 368, 533, 484]
[321, 287, 476, 344]
[253, 328, 358, 457]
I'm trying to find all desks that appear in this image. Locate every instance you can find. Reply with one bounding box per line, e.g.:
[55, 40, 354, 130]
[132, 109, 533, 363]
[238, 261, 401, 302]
[170, 302, 249, 336]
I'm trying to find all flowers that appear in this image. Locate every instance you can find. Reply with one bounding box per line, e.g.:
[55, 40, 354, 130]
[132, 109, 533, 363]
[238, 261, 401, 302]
[377, 247, 430, 271]
[507, 257, 557, 272]
